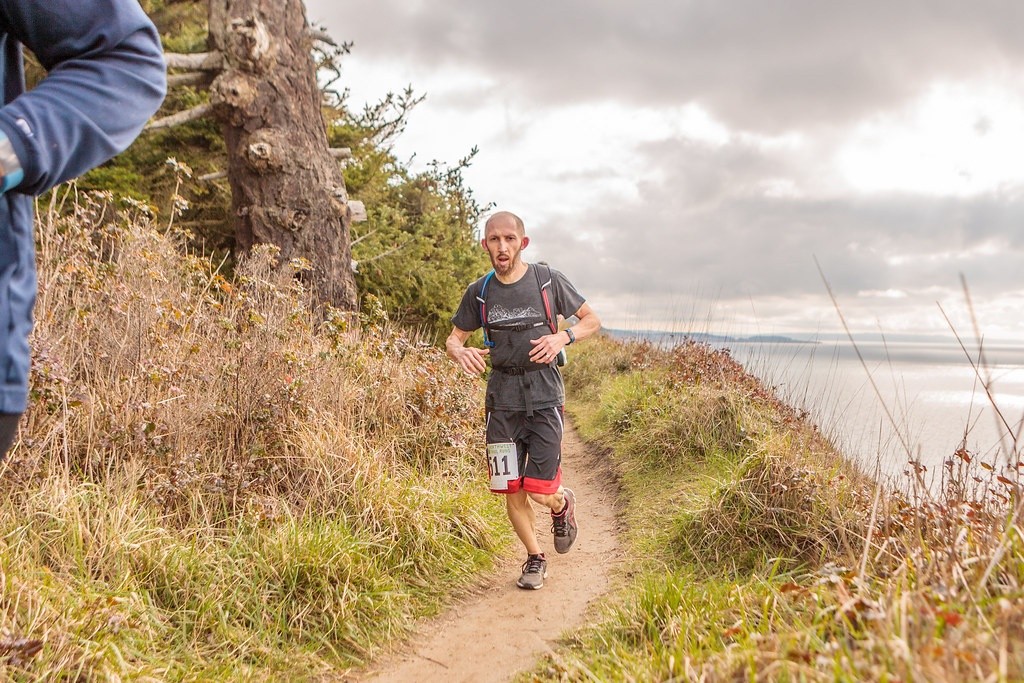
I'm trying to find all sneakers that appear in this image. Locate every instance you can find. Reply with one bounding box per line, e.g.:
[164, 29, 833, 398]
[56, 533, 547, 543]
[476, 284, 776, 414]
[516, 553, 548, 589]
[550, 488, 579, 554]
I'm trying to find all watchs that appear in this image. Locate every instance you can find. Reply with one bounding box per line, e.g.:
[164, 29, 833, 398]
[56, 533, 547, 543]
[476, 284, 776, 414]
[563, 328, 575, 345]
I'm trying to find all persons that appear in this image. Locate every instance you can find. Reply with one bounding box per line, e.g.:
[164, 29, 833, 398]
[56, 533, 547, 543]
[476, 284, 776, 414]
[446, 211, 602, 591]
[0, 0, 168, 453]
